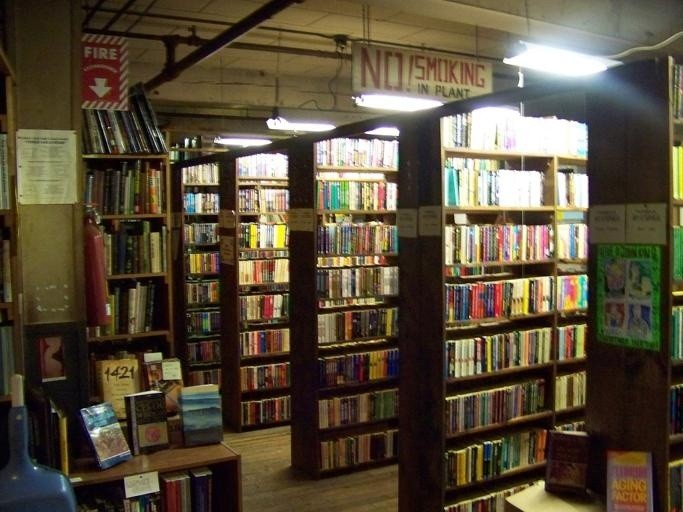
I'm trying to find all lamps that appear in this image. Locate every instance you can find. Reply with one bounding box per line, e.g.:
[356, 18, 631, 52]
[503, 1, 623, 78]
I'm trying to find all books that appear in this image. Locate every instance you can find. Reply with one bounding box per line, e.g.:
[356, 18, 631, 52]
[2, 133, 15, 395]
[670, 63, 683, 512]
[316, 139, 399, 468]
[25, 389, 68, 475]
[183, 163, 221, 390]
[78, 467, 215, 511]
[545, 430, 653, 511]
[80, 350, 223, 469]
[239, 154, 290, 425]
[86, 81, 168, 338]
[442, 108, 587, 511]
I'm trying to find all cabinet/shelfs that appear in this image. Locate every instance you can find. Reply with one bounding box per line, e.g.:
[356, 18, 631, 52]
[80, 128, 174, 427]
[0, 441, 244, 512]
[399, 90, 586, 510]
[289, 124, 398, 481]
[218, 145, 291, 434]
[0, 46, 28, 401]
[173, 155, 223, 386]
[586, 54, 682, 512]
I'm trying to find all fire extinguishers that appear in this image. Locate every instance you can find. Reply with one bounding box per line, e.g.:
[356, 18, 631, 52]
[84, 207, 112, 327]
